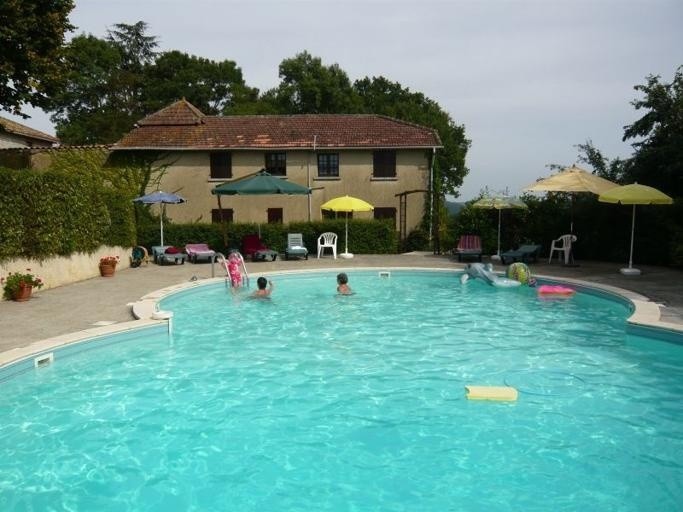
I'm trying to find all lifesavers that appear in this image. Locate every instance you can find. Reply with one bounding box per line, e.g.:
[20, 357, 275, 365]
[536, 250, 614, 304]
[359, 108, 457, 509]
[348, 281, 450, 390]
[461, 263, 521, 287]
[538, 283, 574, 295]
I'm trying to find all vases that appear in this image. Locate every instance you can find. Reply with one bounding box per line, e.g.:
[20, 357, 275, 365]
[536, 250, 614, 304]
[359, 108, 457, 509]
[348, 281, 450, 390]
[101, 265, 115, 277]
[14, 287, 32, 301]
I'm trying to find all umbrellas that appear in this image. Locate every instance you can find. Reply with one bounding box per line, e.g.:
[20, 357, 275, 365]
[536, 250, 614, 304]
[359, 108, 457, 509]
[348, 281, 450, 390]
[521, 165, 622, 261]
[470, 183, 528, 252]
[594, 180, 675, 267]
[131, 189, 187, 246]
[209, 167, 312, 239]
[319, 192, 375, 251]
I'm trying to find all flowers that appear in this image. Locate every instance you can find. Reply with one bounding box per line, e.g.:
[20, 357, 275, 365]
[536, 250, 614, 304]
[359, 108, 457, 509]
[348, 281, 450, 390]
[98, 256, 120, 265]
[1, 269, 44, 296]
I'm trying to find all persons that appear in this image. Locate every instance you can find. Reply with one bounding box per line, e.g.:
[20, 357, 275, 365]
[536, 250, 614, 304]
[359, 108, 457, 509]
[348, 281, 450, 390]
[220, 252, 244, 289]
[231, 277, 275, 299]
[334, 273, 355, 296]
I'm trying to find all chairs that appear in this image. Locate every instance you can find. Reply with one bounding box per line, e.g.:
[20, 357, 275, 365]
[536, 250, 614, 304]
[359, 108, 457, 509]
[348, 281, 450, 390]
[453, 236, 483, 262]
[548, 235, 577, 265]
[286, 233, 308, 260]
[152, 232, 278, 265]
[499, 245, 541, 265]
[317, 232, 338, 259]
